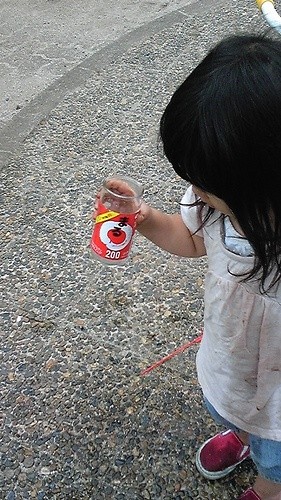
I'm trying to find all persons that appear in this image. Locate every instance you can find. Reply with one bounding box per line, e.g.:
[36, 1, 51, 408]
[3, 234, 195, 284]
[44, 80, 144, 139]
[93, 32, 281, 500]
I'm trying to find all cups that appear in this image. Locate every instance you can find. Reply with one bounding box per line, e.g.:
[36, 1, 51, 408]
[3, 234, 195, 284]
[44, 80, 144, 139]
[91, 175, 143, 265]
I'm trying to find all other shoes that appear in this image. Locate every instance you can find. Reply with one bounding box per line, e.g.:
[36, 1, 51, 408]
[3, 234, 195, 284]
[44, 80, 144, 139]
[239, 487, 262, 500]
[196, 429, 253, 480]
